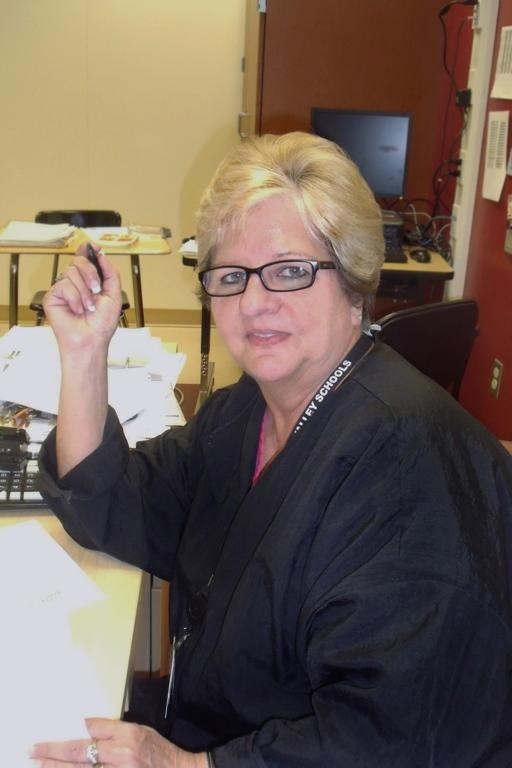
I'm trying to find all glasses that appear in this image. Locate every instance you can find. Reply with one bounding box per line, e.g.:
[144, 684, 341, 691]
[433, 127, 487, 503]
[196, 259, 340, 298]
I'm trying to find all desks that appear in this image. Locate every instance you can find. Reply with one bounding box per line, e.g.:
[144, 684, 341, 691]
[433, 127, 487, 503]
[180, 213, 456, 418]
[1, 384, 201, 767]
[1, 211, 172, 333]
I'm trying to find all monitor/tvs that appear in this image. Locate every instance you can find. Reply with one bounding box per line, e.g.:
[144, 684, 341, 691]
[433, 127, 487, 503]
[311, 106, 413, 200]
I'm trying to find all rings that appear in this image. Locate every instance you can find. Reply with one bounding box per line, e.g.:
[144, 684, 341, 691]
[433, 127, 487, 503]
[84, 736, 101, 765]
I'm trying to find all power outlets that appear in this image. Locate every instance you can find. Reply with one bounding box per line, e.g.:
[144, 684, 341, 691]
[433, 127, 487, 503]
[448, 0, 483, 241]
[488, 357, 504, 399]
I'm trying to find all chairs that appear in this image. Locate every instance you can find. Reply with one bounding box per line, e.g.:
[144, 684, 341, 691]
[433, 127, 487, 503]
[31, 211, 131, 330]
[366, 297, 481, 399]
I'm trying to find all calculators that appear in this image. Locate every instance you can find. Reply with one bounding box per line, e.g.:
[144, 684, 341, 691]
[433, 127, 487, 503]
[1, 440, 60, 515]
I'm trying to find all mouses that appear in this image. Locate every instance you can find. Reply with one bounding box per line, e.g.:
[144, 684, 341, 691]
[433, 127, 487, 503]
[410, 249, 430, 263]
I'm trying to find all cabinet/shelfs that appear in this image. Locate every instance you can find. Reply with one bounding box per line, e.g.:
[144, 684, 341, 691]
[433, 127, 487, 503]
[238, 1, 475, 249]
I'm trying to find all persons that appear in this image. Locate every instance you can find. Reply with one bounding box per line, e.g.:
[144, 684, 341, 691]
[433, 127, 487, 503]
[27, 127, 512, 768]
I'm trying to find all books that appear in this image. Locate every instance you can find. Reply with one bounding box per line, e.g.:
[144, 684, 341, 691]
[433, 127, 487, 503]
[1, 218, 79, 248]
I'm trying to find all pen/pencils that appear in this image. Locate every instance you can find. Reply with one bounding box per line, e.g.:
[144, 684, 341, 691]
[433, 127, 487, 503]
[87, 243, 104, 281]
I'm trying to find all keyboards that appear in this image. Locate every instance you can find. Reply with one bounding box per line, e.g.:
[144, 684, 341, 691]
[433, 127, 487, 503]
[384, 242, 408, 262]
